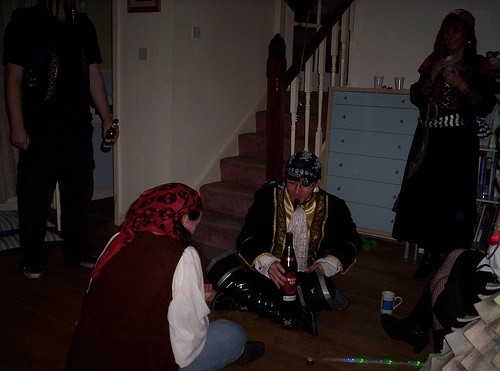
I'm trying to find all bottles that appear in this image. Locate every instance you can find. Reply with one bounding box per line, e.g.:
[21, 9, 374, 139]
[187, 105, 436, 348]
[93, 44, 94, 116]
[446, 55, 455, 74]
[280, 232, 298, 304]
[100, 118, 119, 152]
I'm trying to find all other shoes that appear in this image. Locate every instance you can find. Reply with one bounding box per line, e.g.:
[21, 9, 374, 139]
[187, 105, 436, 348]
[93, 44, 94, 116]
[75, 253, 97, 269]
[412, 247, 442, 280]
[24, 262, 43, 278]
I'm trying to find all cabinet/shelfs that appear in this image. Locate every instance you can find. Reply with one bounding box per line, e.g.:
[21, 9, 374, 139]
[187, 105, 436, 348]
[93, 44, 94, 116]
[328, 87, 418, 242]
[475, 106, 500, 255]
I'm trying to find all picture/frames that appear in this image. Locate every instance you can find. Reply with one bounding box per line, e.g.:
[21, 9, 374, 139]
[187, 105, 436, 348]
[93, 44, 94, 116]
[127, 0, 161, 13]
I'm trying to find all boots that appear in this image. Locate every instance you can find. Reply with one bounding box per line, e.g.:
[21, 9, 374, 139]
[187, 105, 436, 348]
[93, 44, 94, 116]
[212, 269, 334, 313]
[380, 289, 434, 355]
[206, 251, 319, 337]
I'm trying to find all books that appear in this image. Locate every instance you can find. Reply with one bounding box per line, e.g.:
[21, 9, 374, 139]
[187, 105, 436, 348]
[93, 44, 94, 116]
[477, 155, 500, 202]
[473, 105, 500, 250]
[474, 201, 500, 250]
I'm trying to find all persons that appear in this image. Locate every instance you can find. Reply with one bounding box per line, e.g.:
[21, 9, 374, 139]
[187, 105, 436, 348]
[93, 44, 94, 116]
[2, 0, 119, 279]
[66, 183, 265, 371]
[380, 124, 500, 371]
[391, 9, 496, 279]
[207, 151, 363, 336]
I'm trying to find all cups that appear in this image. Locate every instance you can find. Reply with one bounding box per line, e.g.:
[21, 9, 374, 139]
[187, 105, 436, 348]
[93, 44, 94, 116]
[381, 291, 402, 315]
[394, 76, 405, 90]
[373, 76, 384, 89]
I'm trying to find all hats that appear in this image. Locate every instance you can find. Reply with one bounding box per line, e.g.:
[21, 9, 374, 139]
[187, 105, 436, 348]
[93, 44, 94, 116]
[448, 8, 475, 27]
